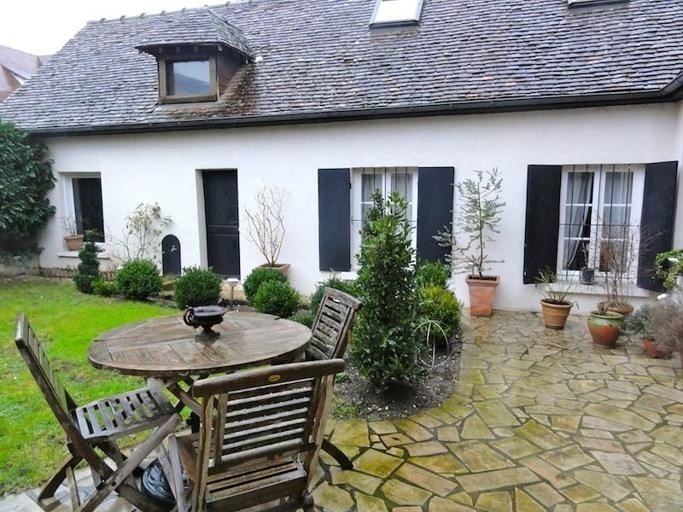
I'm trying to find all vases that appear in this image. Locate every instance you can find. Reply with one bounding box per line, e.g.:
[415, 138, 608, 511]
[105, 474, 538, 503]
[258, 263, 291, 276]
[64, 235, 85, 251]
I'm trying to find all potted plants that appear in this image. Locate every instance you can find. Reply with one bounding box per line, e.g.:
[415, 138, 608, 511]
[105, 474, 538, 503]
[582, 206, 683, 360]
[432, 168, 509, 320]
[522, 264, 582, 331]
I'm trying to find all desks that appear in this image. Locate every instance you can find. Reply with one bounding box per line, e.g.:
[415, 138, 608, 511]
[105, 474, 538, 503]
[84, 306, 317, 509]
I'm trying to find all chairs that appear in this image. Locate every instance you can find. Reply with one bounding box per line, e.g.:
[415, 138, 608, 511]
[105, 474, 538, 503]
[161, 356, 348, 512]
[274, 287, 363, 477]
[15, 310, 180, 512]
